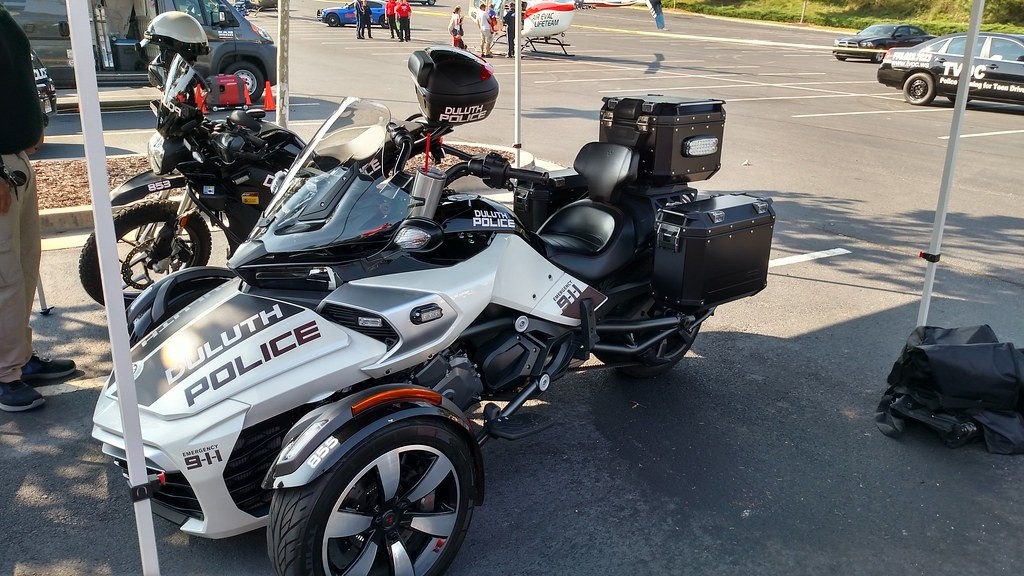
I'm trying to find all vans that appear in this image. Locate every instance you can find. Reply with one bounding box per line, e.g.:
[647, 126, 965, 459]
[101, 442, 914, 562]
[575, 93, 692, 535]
[0, 0, 277, 104]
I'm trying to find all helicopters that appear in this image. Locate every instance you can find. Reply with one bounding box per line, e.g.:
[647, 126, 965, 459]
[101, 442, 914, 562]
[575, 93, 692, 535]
[469, 0, 670, 59]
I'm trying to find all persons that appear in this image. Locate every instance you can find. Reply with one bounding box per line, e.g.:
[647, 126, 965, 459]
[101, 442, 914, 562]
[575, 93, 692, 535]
[452, 1, 527, 57]
[0, 4, 76, 412]
[354, 0, 412, 42]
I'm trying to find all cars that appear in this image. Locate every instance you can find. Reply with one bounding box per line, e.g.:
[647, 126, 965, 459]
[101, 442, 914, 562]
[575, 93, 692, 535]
[407, 0, 436, 6]
[831, 24, 940, 64]
[877, 32, 1024, 105]
[317, 0, 389, 29]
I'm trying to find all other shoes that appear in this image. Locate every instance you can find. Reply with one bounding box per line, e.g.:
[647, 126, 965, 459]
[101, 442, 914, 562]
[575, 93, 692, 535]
[481, 54, 494, 58]
[391, 36, 394, 38]
[357, 37, 363, 39]
[505, 54, 513, 58]
[369, 37, 372, 38]
[399, 40, 403, 42]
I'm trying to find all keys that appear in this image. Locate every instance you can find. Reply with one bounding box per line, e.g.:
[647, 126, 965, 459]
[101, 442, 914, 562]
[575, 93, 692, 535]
[3, 163, 26, 201]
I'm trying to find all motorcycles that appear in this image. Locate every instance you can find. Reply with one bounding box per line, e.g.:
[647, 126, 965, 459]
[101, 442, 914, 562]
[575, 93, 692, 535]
[78, 11, 315, 309]
[91, 47, 776, 576]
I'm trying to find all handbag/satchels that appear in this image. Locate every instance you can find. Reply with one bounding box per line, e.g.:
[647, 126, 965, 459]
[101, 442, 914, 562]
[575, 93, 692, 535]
[448, 17, 454, 35]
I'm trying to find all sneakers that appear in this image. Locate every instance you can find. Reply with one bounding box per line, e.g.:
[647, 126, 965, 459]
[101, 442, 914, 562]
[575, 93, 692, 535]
[0, 377, 44, 412]
[20, 354, 75, 379]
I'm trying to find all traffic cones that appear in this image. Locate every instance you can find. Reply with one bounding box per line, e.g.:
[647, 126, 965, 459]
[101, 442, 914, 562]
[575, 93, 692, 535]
[193, 84, 210, 115]
[241, 78, 252, 106]
[263, 81, 277, 112]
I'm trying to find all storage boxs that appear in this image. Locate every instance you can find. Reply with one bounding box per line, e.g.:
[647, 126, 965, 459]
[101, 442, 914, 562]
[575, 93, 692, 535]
[598, 94, 725, 175]
[512, 169, 594, 232]
[112, 39, 141, 70]
[407, 46, 499, 125]
[647, 194, 775, 307]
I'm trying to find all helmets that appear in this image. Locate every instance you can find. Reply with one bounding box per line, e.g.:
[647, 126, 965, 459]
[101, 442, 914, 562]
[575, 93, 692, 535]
[144, 12, 211, 57]
